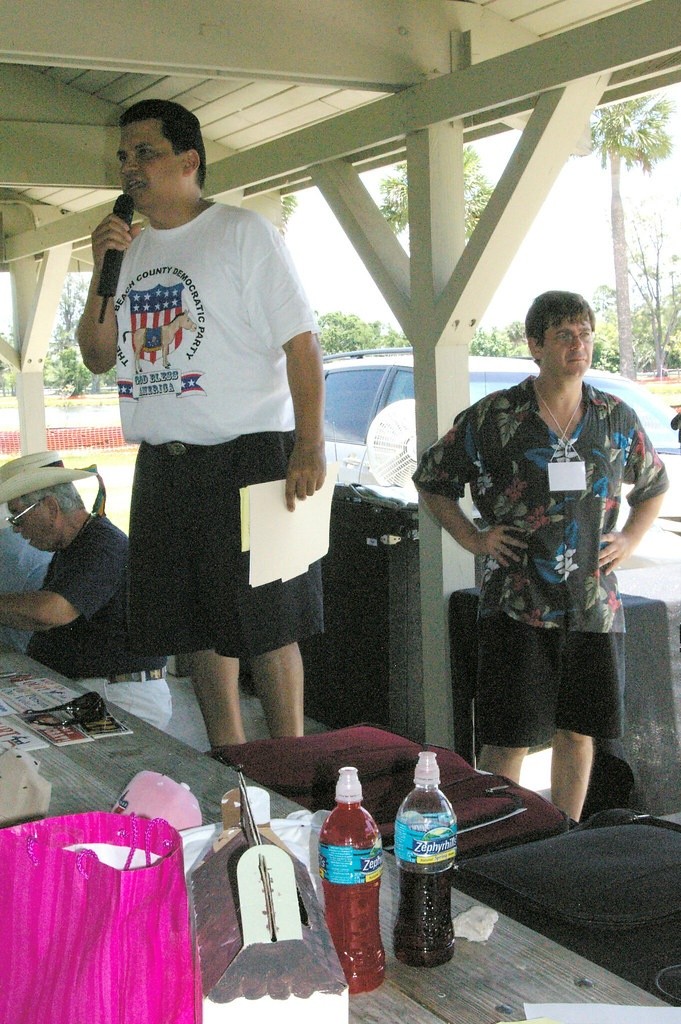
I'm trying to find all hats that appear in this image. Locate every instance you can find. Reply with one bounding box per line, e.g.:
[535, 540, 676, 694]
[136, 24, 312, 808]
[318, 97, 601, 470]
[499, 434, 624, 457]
[0, 450, 106, 517]
[670, 400, 679, 407]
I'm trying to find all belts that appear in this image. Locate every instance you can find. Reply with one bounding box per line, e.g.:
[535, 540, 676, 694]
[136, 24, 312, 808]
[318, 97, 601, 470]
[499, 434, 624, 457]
[108, 666, 167, 685]
[158, 441, 186, 458]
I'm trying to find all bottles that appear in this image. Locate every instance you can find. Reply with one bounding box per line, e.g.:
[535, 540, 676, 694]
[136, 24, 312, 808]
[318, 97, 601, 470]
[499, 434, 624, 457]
[394, 751, 455, 967]
[322, 764, 386, 994]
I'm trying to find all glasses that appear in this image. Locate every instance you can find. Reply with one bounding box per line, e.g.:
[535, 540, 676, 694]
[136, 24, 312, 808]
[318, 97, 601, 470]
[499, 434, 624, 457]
[5, 496, 48, 526]
[542, 330, 598, 343]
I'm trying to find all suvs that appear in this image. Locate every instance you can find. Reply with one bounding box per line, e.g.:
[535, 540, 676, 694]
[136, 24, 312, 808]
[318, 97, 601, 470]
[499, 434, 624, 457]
[321, 346, 681, 534]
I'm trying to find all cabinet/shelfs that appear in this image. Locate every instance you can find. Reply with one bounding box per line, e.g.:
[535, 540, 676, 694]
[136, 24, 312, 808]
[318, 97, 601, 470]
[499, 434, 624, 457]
[236, 501, 425, 743]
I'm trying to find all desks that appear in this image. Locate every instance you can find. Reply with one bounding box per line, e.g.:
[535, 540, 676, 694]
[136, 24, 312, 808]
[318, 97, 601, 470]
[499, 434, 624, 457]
[0, 648, 674, 1024]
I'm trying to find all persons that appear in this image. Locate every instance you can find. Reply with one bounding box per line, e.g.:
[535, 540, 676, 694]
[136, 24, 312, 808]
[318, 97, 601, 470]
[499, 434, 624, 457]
[74, 100, 331, 753]
[0, 450, 175, 731]
[411, 290, 669, 824]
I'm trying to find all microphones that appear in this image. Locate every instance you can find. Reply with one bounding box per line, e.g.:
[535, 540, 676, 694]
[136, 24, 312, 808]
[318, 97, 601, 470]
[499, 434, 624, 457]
[96, 193, 134, 324]
